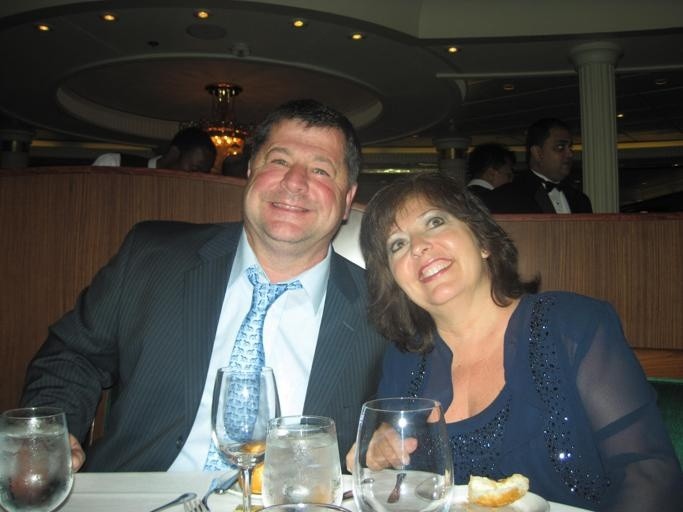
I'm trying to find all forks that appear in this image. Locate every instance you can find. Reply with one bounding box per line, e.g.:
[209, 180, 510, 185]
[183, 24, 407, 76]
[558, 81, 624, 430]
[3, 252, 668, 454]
[183, 469, 240, 512]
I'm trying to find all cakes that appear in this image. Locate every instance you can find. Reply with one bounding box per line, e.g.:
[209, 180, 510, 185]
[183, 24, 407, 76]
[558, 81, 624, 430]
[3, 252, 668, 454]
[468, 473, 528, 508]
[238, 441, 267, 495]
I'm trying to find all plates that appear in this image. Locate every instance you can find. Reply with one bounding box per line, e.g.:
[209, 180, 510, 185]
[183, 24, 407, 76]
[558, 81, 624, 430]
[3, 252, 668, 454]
[456, 483, 551, 512]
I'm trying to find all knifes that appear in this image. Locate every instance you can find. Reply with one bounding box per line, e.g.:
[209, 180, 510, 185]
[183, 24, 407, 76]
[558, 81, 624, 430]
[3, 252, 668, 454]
[388, 472, 407, 503]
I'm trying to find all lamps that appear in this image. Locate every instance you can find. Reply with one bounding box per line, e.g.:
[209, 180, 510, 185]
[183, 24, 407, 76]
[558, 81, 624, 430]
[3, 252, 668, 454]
[193, 76, 254, 159]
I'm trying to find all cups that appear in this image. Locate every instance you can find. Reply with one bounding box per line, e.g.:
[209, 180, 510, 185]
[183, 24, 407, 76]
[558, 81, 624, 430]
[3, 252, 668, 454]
[0, 407, 73, 512]
[352, 398, 453, 512]
[210, 365, 280, 512]
[264, 416, 342, 509]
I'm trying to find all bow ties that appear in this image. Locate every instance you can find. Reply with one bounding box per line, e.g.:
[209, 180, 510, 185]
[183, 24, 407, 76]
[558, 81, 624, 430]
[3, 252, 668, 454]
[544, 182, 566, 193]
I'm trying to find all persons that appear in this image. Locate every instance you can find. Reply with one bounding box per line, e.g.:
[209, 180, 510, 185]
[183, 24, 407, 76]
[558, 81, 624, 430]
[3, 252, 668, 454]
[457, 141, 517, 215]
[15, 100, 400, 472]
[91, 125, 216, 174]
[482, 116, 588, 218]
[348, 172, 683, 510]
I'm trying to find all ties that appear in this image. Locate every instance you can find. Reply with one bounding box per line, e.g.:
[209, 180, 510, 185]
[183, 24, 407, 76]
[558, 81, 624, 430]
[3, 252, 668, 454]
[205, 267, 303, 472]
